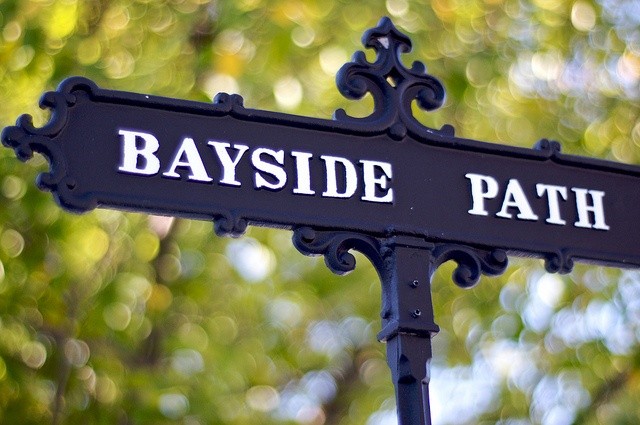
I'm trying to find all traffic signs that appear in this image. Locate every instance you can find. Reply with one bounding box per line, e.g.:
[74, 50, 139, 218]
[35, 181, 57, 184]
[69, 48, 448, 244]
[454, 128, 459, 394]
[6, 76, 639, 265]
